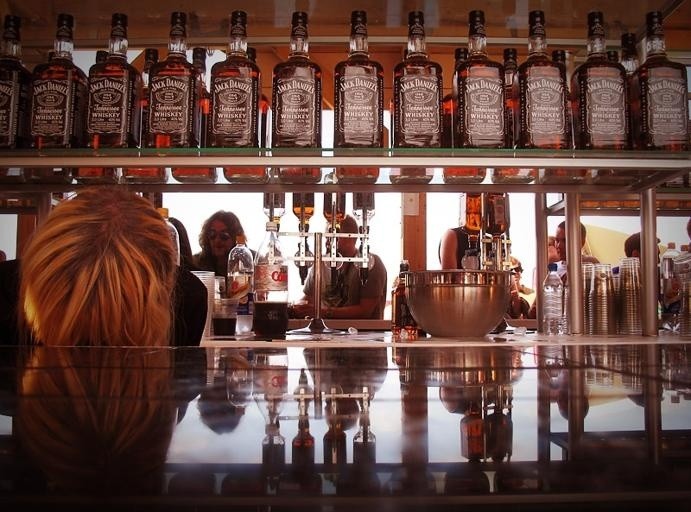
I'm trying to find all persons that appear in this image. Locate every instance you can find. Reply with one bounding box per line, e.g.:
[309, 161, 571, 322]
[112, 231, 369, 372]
[438, 191, 530, 335]
[289, 212, 388, 320]
[16, 182, 178, 348]
[190, 211, 271, 303]
[525, 218, 691, 332]
[20, 347, 175, 494]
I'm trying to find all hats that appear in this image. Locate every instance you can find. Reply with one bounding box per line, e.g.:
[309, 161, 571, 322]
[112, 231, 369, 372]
[624, 232, 660, 254]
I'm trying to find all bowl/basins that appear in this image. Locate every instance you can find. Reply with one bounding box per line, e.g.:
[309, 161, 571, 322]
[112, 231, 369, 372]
[404, 342, 513, 388]
[404, 267, 511, 340]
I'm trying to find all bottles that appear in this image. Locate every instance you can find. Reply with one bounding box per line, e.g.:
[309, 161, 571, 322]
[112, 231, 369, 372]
[391, 260, 417, 341]
[227, 344, 288, 435]
[542, 259, 563, 337]
[228, 234, 290, 342]
[541, 346, 566, 390]
[459, 193, 511, 251]
[262, 192, 377, 285]
[0, 8, 689, 184]
[461, 406, 513, 463]
[661, 241, 691, 339]
[262, 399, 378, 477]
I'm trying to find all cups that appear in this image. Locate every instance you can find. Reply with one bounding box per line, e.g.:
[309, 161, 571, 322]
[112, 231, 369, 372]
[582, 256, 642, 341]
[191, 268, 213, 340]
[214, 297, 237, 339]
[585, 346, 691, 391]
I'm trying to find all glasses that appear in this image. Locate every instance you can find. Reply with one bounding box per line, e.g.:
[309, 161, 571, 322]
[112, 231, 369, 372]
[205, 228, 231, 241]
